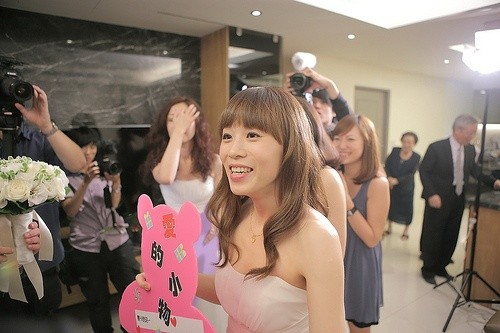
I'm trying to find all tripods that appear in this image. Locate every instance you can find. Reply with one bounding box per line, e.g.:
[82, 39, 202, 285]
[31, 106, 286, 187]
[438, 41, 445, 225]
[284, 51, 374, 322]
[433, 91, 500, 333]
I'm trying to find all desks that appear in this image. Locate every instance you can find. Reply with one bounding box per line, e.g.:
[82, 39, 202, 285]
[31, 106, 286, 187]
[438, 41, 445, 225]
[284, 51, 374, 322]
[462, 190, 500, 311]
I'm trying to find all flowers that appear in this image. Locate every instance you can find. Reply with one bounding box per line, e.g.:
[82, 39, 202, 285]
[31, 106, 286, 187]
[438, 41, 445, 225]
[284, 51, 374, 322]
[0, 156, 72, 303]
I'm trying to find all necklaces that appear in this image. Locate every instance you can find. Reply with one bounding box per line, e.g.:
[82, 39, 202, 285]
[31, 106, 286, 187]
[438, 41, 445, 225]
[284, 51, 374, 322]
[249, 209, 264, 243]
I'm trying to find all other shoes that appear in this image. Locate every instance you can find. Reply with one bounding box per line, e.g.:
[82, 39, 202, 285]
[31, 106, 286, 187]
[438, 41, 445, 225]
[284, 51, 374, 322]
[382, 229, 392, 236]
[435, 269, 454, 280]
[402, 231, 408, 241]
[422, 267, 437, 284]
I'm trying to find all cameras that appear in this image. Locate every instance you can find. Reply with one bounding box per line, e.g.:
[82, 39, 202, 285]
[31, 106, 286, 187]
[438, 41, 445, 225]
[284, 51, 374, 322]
[0, 69, 34, 130]
[289, 73, 310, 96]
[96, 158, 121, 177]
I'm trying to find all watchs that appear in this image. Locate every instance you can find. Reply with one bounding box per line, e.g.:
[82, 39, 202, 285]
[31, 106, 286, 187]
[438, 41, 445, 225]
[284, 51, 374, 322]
[347, 206, 358, 216]
[40, 121, 58, 136]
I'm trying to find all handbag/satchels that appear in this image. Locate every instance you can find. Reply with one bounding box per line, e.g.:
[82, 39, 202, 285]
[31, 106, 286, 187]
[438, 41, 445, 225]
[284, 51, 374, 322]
[59, 238, 88, 288]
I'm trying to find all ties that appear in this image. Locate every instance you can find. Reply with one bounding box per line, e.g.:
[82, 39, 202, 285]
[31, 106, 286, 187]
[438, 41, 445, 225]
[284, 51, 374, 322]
[455, 145, 463, 196]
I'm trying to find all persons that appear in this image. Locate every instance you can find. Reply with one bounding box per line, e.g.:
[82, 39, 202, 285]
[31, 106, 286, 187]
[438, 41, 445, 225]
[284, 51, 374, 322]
[331, 114, 390, 333]
[61, 127, 140, 333]
[134, 87, 348, 333]
[284, 67, 351, 136]
[385, 132, 420, 240]
[141, 96, 229, 333]
[0, 85, 86, 316]
[418, 114, 500, 285]
